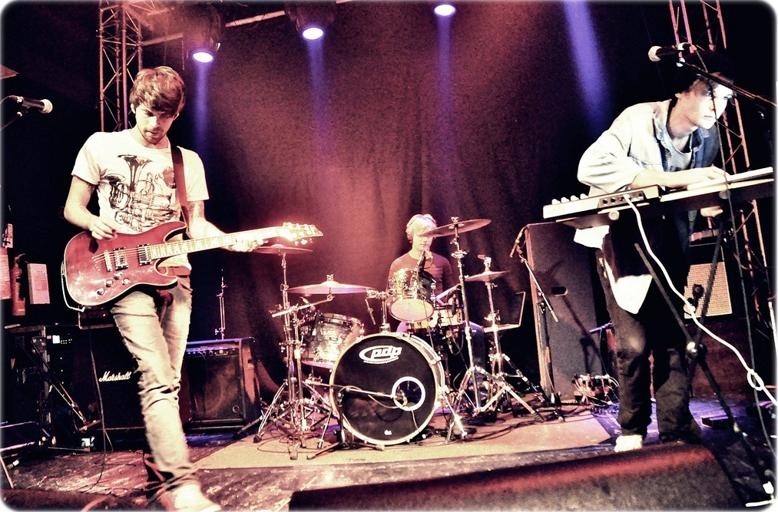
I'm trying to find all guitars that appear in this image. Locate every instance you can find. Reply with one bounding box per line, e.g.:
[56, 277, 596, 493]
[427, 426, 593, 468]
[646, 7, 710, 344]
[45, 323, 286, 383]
[64, 220, 323, 308]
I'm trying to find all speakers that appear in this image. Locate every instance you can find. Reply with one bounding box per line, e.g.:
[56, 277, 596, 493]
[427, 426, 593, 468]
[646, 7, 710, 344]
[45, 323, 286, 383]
[53, 322, 143, 447]
[177, 336, 250, 433]
[523, 221, 604, 400]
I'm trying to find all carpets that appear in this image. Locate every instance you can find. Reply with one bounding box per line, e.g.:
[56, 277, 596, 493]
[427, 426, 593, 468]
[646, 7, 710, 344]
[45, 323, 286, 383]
[195, 397, 613, 469]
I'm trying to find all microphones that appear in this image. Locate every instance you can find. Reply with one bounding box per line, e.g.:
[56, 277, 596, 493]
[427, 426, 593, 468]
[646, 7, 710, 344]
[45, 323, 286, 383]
[648, 43, 689, 62]
[17, 96, 54, 113]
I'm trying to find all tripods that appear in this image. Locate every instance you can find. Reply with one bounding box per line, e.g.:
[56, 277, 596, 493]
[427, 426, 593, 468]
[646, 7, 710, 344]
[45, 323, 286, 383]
[307, 415, 385, 461]
[377, 280, 564, 421]
[253, 282, 339, 461]
[444, 240, 544, 443]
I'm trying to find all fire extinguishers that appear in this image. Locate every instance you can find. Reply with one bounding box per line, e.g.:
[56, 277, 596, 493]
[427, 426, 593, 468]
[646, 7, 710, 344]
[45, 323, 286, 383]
[11, 253, 26, 316]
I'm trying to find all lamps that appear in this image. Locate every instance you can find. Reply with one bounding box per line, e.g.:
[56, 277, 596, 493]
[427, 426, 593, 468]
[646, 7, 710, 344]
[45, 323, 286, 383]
[184, 0, 223, 64]
[281, 0, 334, 42]
[429, 0, 456, 16]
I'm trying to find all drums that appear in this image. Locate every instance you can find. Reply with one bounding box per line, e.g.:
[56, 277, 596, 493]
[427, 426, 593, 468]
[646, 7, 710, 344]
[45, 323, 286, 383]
[406, 302, 464, 335]
[300, 312, 365, 369]
[330, 332, 444, 444]
[387, 266, 436, 323]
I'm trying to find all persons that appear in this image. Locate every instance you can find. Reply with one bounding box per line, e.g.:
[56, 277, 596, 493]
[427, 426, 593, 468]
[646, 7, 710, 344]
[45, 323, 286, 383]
[573, 68, 739, 452]
[388, 213, 486, 403]
[62, 66, 267, 512]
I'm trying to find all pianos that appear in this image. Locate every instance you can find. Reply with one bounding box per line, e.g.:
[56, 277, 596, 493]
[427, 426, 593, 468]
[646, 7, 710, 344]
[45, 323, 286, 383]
[542, 167, 775, 223]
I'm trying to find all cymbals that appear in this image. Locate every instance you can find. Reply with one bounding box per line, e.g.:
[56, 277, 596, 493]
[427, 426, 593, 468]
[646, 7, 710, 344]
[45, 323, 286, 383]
[419, 217, 491, 237]
[287, 281, 374, 295]
[465, 270, 510, 282]
[478, 322, 519, 333]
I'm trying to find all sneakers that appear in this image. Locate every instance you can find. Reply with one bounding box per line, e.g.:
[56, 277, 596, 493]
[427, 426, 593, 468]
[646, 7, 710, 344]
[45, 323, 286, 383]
[613, 432, 644, 455]
[146, 480, 222, 512]
